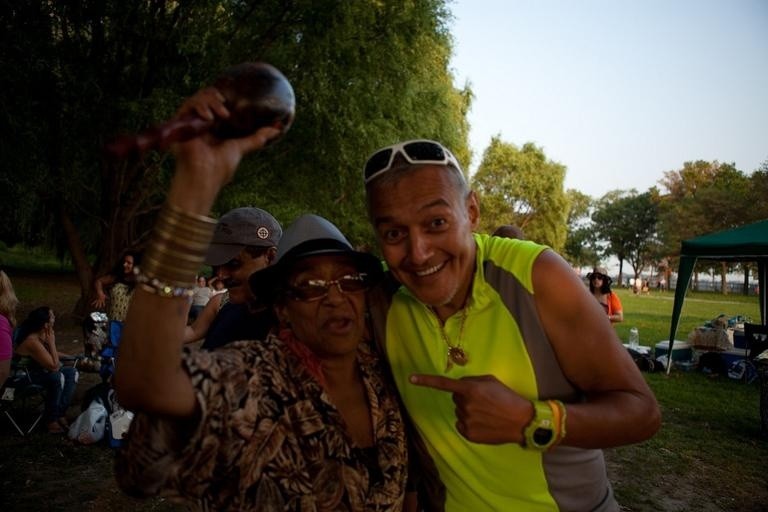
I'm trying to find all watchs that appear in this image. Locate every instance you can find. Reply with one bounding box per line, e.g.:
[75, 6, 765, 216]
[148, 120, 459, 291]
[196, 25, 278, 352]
[523, 400, 555, 451]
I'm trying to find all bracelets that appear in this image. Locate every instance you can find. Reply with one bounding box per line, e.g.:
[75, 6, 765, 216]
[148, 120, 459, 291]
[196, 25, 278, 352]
[608, 316, 611, 320]
[546, 399, 567, 444]
[135, 200, 217, 299]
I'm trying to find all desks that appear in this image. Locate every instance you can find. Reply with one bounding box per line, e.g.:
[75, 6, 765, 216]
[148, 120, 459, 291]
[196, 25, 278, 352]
[687, 323, 744, 353]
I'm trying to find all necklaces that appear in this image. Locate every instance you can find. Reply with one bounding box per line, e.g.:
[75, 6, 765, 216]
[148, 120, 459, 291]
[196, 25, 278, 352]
[428, 301, 472, 374]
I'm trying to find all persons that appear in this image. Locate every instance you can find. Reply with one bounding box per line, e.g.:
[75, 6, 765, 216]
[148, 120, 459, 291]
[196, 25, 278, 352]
[112, 87, 410, 511]
[12, 306, 80, 446]
[585, 267, 623, 327]
[366, 138, 662, 512]
[179, 291, 231, 346]
[206, 276, 231, 312]
[0, 270, 23, 390]
[191, 276, 210, 316]
[196, 206, 283, 353]
[490, 225, 526, 241]
[90, 251, 144, 364]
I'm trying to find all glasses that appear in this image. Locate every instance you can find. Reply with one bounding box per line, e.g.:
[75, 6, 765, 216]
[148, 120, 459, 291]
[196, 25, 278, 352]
[591, 274, 602, 281]
[284, 270, 371, 303]
[363, 137, 469, 183]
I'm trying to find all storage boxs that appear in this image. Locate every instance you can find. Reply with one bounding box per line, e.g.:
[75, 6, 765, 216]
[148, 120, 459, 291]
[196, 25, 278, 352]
[654, 338, 692, 369]
[733, 330, 745, 349]
[720, 346, 751, 378]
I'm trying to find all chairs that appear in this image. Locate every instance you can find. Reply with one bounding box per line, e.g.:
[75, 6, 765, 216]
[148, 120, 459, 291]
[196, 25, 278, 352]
[0, 363, 44, 438]
[742, 321, 768, 386]
[10, 327, 85, 434]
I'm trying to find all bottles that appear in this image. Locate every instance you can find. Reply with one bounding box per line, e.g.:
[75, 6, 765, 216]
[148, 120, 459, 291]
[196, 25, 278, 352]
[629, 327, 639, 347]
[104, 440, 127, 492]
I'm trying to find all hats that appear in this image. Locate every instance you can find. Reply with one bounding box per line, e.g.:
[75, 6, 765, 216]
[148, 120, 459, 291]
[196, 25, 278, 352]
[250, 213, 381, 301]
[586, 267, 612, 281]
[204, 206, 281, 267]
